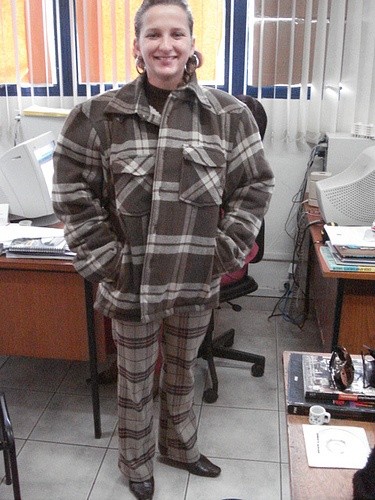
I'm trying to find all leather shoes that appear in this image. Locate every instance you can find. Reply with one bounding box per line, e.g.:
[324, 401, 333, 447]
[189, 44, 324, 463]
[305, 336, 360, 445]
[161, 452, 221, 477]
[129, 476, 154, 500]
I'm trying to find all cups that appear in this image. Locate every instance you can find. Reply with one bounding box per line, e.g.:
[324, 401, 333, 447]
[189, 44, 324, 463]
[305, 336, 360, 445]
[309, 405, 330, 424]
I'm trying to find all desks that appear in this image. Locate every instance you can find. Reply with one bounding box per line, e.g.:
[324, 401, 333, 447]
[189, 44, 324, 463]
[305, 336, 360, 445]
[283, 351, 375, 499]
[302, 194, 375, 355]
[0, 218, 112, 439]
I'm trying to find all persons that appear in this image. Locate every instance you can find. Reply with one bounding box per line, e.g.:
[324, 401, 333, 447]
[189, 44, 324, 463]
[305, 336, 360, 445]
[51, 0, 275, 500]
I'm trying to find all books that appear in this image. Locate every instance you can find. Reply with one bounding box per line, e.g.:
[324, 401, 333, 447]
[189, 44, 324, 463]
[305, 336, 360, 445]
[318, 221, 375, 272]
[3, 221, 78, 260]
[286, 351, 375, 423]
[22, 103, 72, 118]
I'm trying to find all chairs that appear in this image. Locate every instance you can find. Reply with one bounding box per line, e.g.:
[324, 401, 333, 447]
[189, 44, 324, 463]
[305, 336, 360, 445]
[195, 217, 266, 404]
